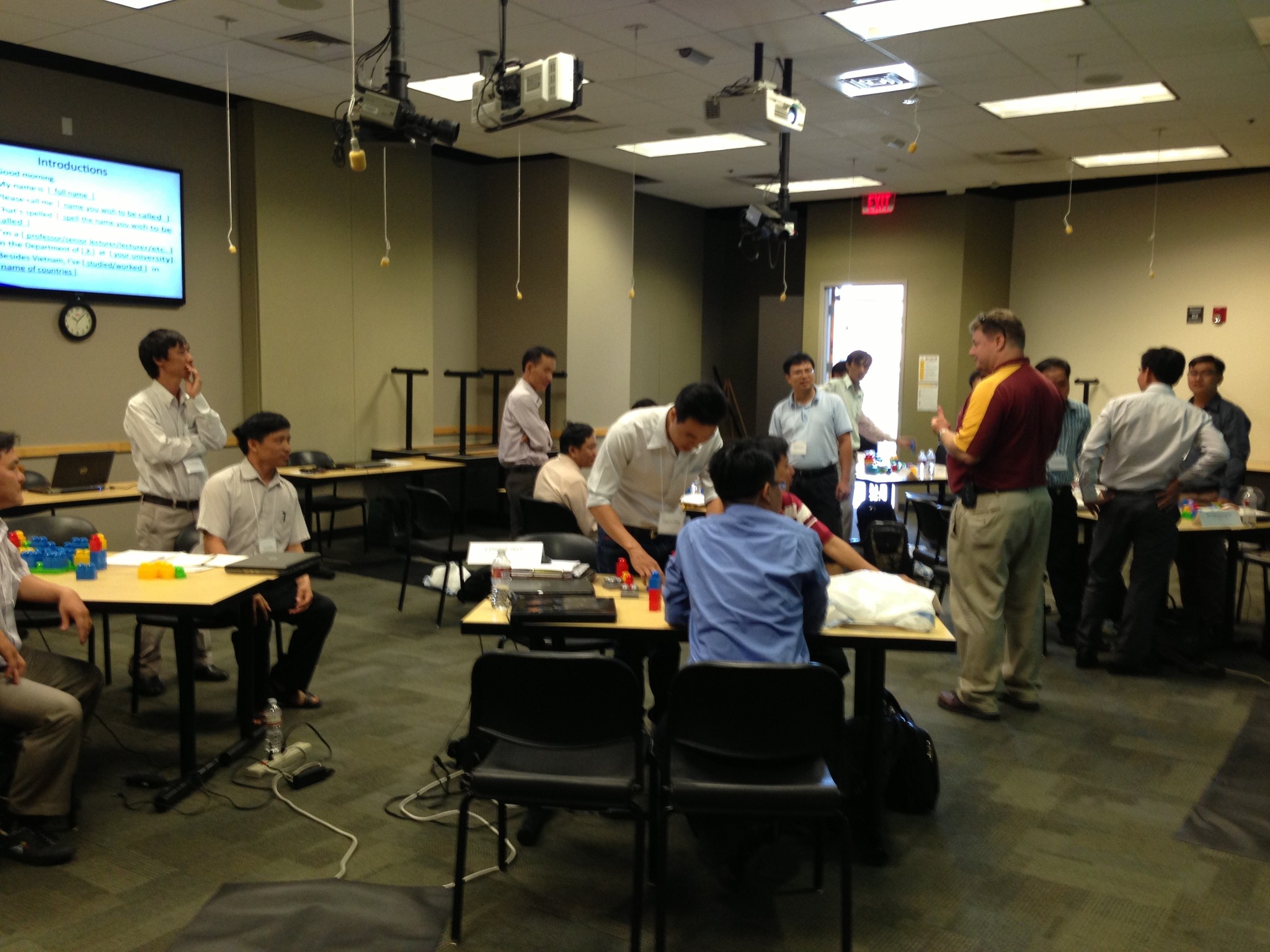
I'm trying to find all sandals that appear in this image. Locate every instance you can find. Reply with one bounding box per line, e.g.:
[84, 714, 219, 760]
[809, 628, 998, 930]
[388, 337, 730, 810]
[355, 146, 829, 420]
[281, 688, 319, 708]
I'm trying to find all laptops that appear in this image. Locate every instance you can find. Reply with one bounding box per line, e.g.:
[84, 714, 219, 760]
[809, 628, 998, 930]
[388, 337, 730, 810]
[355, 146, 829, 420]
[507, 575, 595, 605]
[225, 552, 321, 575]
[49, 451, 115, 493]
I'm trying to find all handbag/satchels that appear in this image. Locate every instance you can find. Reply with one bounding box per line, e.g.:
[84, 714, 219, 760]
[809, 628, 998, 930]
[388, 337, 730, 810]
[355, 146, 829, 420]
[842, 687, 941, 815]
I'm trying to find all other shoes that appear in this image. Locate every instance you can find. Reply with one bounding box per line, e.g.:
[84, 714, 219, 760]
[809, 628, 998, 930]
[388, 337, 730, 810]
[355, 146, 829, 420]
[1061, 628, 1077, 643]
[1, 818, 72, 866]
[936, 691, 999, 720]
[996, 689, 1040, 712]
[1075, 652, 1109, 668]
[29, 811, 75, 836]
[1108, 660, 1158, 677]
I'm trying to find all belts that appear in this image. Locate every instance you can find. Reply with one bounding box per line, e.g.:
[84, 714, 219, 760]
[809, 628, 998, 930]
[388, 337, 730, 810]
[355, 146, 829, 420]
[141, 494, 198, 512]
[1110, 487, 1161, 497]
[956, 485, 992, 497]
[793, 465, 837, 477]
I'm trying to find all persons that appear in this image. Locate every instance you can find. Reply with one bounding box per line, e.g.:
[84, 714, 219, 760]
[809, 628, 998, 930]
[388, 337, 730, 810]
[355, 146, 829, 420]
[586, 381, 731, 586]
[0, 431, 103, 864]
[1173, 355, 1252, 677]
[821, 350, 872, 543]
[196, 411, 338, 729]
[1075, 347, 1231, 674]
[123, 329, 228, 694]
[767, 351, 853, 566]
[533, 422, 599, 542]
[970, 370, 985, 391]
[497, 345, 556, 540]
[1033, 358, 1091, 645]
[661, 440, 832, 667]
[753, 437, 918, 585]
[831, 361, 896, 444]
[930, 307, 1068, 720]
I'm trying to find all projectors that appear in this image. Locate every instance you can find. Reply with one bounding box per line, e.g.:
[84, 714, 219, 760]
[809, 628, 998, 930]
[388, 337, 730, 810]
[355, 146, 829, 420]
[718, 89, 807, 135]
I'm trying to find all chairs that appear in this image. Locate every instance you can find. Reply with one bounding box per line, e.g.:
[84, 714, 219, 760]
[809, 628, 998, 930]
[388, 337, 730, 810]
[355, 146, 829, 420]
[902, 498, 952, 602]
[284, 447, 368, 573]
[902, 442, 952, 547]
[512, 530, 602, 574]
[653, 658, 861, 952]
[443, 647, 647, 952]
[121, 609, 288, 734]
[4, 469, 116, 687]
[397, 479, 486, 626]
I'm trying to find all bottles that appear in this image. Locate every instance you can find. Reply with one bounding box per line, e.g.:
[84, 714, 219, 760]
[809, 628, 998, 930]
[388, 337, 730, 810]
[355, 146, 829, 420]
[916, 447, 936, 480]
[1242, 486, 1256, 528]
[491, 549, 512, 610]
[264, 698, 282, 754]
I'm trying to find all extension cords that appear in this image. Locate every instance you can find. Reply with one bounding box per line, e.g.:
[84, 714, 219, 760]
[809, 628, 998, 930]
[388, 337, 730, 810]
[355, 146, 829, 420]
[246, 741, 311, 778]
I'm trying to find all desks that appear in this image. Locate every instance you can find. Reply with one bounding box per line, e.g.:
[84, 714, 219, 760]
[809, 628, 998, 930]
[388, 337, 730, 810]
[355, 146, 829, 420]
[0, 365, 1270, 952]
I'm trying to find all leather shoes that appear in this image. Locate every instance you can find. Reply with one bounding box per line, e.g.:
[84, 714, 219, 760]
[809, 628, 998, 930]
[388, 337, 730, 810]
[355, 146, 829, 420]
[193, 662, 230, 682]
[128, 668, 166, 696]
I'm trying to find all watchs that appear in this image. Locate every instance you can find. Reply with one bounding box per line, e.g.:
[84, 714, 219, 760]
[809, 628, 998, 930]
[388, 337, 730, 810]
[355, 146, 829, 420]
[938, 429, 950, 441]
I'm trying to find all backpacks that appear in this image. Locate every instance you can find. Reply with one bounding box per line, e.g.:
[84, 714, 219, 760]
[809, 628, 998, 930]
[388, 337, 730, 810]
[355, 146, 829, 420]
[862, 519, 912, 574]
